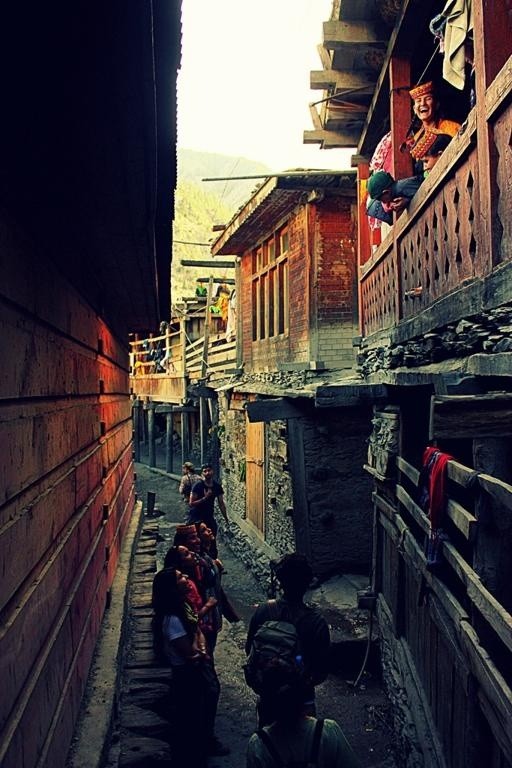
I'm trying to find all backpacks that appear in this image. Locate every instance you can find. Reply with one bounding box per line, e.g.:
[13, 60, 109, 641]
[243, 599, 311, 699]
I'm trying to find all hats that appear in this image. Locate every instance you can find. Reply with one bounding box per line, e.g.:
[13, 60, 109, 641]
[367, 171, 391, 199]
[182, 462, 195, 470]
[409, 81, 433, 99]
[409, 130, 437, 157]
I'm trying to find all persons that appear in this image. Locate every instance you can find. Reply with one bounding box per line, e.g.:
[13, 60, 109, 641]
[410, 128, 453, 174]
[195, 282, 207, 305]
[409, 80, 462, 138]
[245, 552, 358, 768]
[367, 171, 425, 211]
[151, 460, 239, 767]
